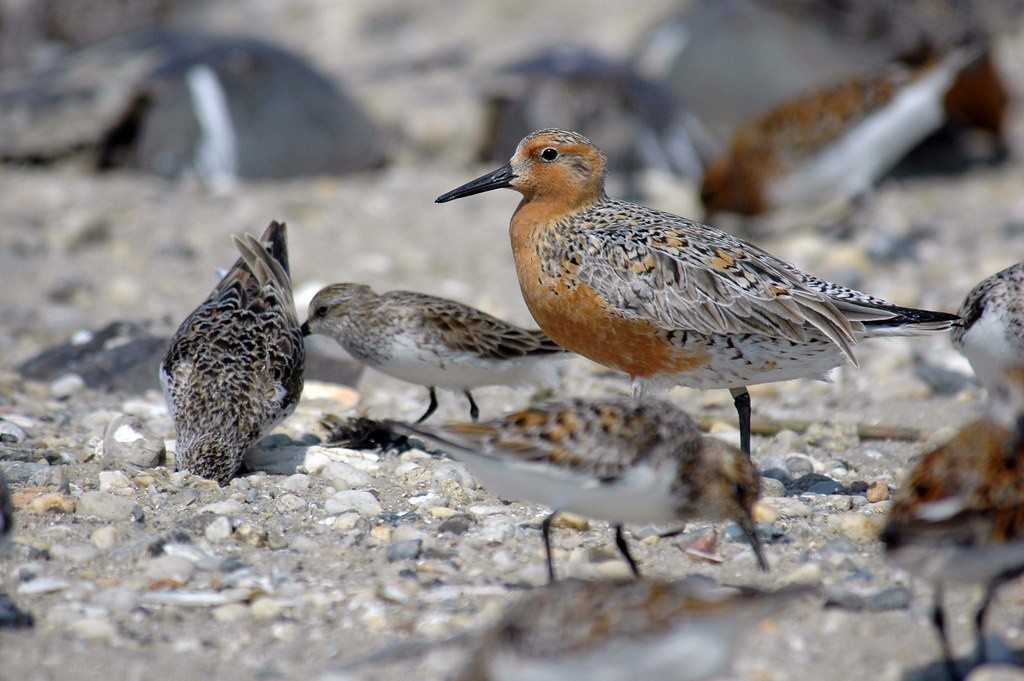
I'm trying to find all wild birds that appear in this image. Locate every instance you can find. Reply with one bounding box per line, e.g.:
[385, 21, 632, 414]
[949, 261, 1024, 417]
[435, 129, 964, 464]
[701, 39, 1008, 234]
[878, 418, 1024, 681]
[379, 397, 770, 588]
[160, 220, 566, 483]
